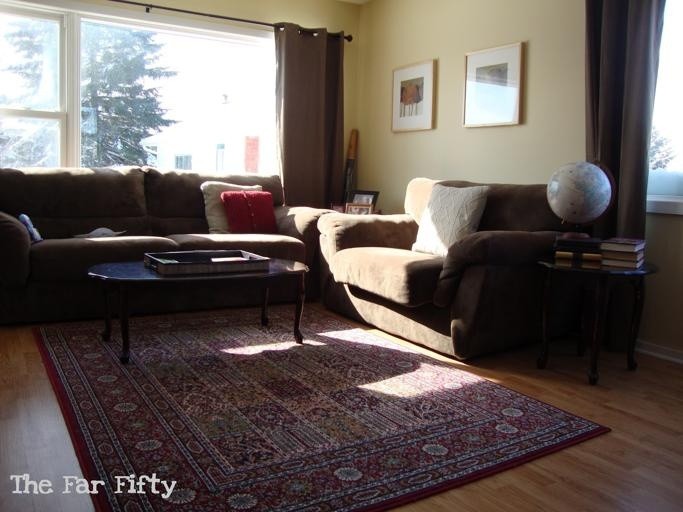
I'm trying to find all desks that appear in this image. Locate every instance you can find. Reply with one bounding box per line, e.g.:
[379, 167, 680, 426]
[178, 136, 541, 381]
[535, 256, 657, 386]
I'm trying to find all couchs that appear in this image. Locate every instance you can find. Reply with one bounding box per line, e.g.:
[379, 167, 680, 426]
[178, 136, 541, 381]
[0, 164, 326, 326]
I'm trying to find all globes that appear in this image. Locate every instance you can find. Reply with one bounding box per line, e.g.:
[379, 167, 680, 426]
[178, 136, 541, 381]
[546, 160, 617, 239]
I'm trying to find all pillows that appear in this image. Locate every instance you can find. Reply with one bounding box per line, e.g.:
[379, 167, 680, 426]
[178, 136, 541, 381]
[412, 184, 490, 257]
[200, 180, 277, 234]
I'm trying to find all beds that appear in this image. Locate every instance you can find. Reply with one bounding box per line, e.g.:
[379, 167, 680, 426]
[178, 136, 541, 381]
[88, 255, 311, 364]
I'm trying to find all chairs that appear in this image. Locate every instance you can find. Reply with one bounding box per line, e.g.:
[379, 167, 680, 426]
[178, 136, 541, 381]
[316, 181, 577, 361]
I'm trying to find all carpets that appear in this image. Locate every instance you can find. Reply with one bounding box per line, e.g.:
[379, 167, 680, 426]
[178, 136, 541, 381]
[31, 303, 611, 512]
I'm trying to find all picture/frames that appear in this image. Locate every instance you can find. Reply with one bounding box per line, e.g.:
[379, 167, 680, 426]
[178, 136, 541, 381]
[331, 190, 379, 214]
[461, 42, 524, 129]
[391, 59, 436, 133]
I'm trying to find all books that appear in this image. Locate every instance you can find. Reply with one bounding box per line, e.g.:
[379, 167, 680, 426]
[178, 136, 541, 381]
[554, 234, 647, 270]
